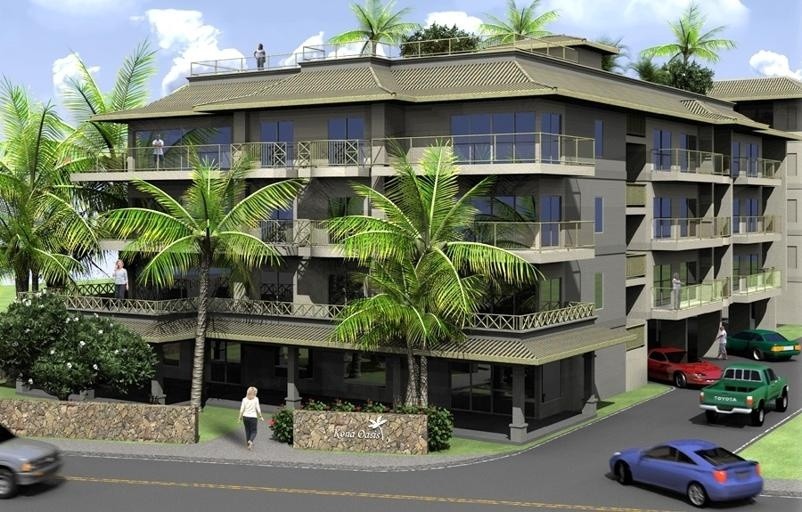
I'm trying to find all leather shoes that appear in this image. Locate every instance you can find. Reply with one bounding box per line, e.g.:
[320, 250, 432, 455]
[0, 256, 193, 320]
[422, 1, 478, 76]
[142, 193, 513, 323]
[247, 440, 253, 451]
